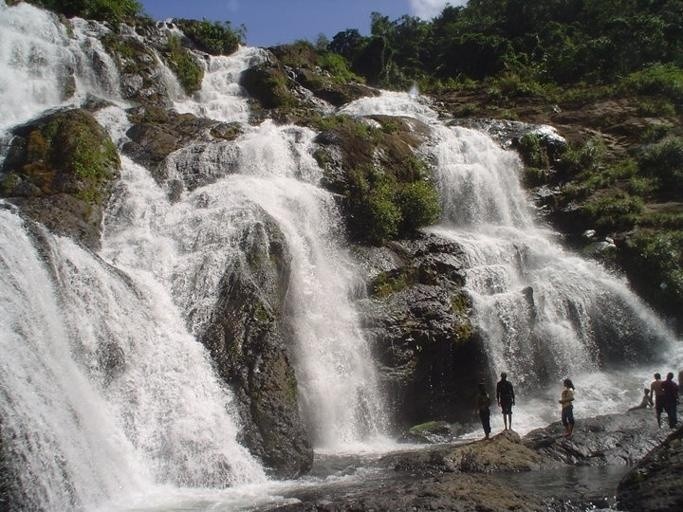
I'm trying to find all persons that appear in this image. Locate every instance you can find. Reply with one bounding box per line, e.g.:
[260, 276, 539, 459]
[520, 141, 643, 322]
[661, 373, 679, 429]
[559, 379, 575, 437]
[496, 372, 515, 430]
[473, 383, 491, 440]
[649, 373, 670, 428]
[628, 388, 653, 411]
